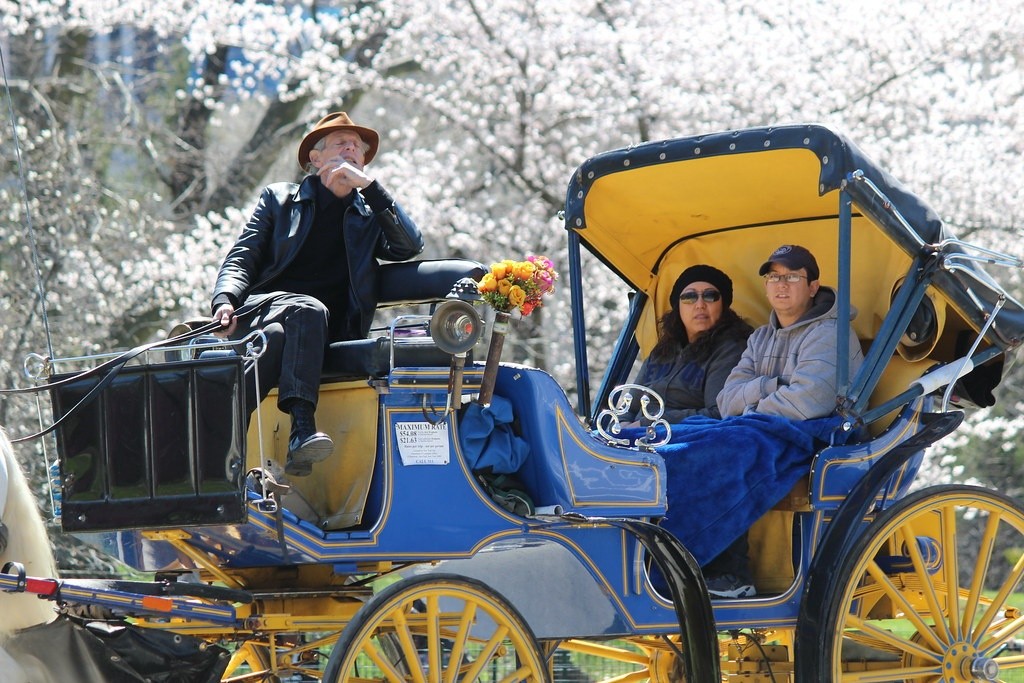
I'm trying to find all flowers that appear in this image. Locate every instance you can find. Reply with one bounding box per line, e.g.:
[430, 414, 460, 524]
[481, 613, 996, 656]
[481, 256, 557, 314]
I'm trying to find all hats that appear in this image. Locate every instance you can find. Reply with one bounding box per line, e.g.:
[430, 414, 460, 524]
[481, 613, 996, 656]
[759, 245, 819, 280]
[298, 112, 380, 173]
[669, 265, 733, 310]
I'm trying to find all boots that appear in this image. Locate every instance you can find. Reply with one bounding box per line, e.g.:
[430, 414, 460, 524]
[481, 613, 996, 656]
[284, 399, 335, 477]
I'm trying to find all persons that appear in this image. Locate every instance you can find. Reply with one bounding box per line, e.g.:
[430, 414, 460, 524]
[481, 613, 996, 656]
[608, 264, 755, 432]
[211, 111, 423, 477]
[709, 245, 868, 598]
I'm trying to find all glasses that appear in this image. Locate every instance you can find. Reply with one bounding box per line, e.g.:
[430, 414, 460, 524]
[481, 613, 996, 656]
[679, 290, 721, 304]
[763, 272, 807, 282]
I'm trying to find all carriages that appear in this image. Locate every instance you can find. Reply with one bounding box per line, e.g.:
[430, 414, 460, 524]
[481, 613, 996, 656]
[0, 123, 1024, 683]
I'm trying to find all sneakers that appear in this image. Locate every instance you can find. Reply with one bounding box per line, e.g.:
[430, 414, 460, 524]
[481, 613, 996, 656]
[706, 574, 757, 599]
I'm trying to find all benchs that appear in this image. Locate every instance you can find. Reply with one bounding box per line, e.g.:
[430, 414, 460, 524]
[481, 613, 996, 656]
[475, 359, 668, 518]
[747, 355, 947, 598]
[204, 261, 491, 383]
